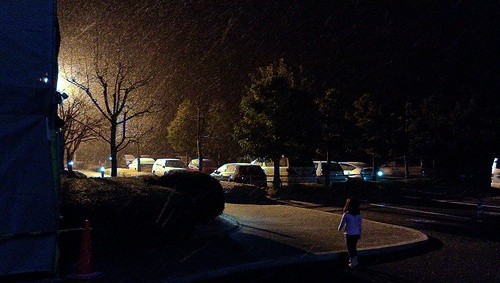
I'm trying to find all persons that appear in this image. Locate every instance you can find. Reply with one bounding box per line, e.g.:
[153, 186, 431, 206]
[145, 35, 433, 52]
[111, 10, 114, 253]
[338, 198, 361, 269]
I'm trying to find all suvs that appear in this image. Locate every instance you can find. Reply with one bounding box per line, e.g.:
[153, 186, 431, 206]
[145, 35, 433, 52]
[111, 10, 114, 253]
[249, 156, 320, 183]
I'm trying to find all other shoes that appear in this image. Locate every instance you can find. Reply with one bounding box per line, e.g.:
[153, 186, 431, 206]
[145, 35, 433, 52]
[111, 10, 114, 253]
[349, 256, 357, 267]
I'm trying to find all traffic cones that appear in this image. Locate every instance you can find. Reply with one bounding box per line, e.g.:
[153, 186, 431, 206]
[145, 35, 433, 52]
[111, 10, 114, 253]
[66, 220, 106, 281]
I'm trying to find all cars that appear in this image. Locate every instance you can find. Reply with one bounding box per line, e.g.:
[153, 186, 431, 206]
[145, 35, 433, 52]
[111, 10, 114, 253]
[128, 157, 155, 173]
[313, 161, 345, 180]
[188, 158, 219, 174]
[151, 158, 187, 177]
[208, 162, 267, 189]
[338, 161, 374, 180]
[490, 157, 500, 189]
[377, 157, 424, 179]
